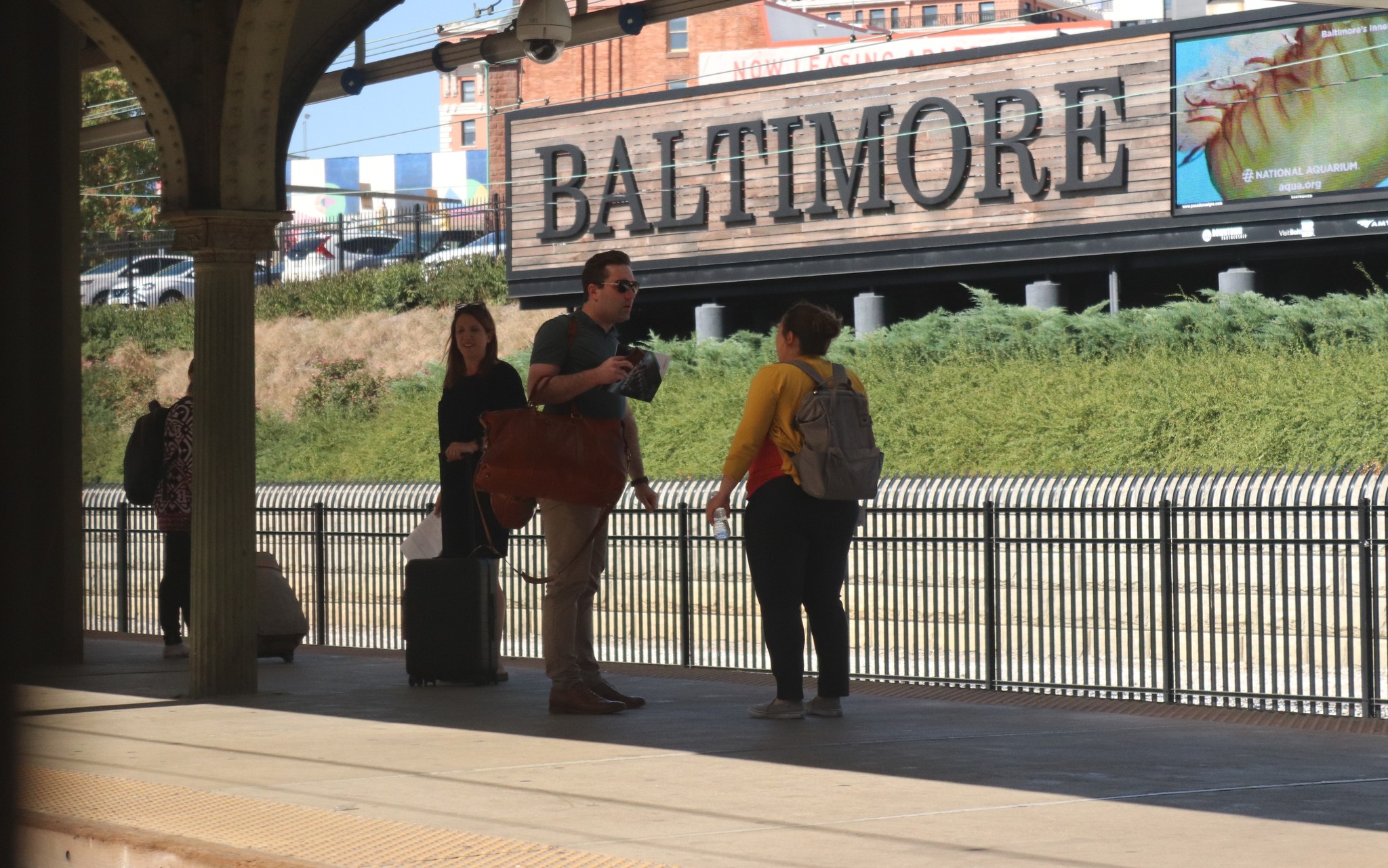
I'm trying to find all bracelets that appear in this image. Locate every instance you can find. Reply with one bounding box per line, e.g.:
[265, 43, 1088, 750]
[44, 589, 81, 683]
[630, 476, 648, 487]
[467, 437, 483, 457]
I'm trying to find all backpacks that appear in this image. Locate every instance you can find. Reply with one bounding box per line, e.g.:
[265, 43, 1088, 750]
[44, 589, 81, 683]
[778, 359, 884, 502]
[124, 397, 193, 505]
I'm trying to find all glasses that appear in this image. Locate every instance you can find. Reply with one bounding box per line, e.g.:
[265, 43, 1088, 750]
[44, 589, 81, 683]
[454, 302, 488, 318]
[601, 279, 640, 295]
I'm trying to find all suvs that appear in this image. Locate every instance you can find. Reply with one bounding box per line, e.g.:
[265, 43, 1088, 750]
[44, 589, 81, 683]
[353, 230, 492, 273]
[281, 232, 427, 287]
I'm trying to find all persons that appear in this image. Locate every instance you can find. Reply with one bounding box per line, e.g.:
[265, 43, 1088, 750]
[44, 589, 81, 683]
[433, 303, 526, 685]
[706, 301, 870, 718]
[152, 358, 195, 656]
[527, 249, 660, 715]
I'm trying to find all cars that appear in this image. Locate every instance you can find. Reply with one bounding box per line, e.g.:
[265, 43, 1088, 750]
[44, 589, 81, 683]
[420, 230, 506, 281]
[81, 255, 285, 311]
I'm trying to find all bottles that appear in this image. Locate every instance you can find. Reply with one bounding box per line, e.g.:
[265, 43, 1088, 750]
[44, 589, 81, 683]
[710, 490, 731, 540]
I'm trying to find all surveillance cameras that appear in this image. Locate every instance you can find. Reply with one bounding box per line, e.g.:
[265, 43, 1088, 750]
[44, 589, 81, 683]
[516, 0, 572, 64]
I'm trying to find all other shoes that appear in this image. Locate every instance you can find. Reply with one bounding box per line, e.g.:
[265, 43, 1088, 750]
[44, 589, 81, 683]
[588, 681, 647, 709]
[476, 670, 511, 685]
[549, 683, 627, 716]
[163, 643, 191, 660]
[801, 697, 846, 719]
[748, 695, 803, 720]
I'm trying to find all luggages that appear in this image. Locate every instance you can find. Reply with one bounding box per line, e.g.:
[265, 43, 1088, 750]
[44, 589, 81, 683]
[249, 551, 308, 662]
[400, 453, 490, 689]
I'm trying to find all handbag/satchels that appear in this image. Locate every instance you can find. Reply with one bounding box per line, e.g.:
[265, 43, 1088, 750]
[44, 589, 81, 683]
[477, 374, 631, 501]
